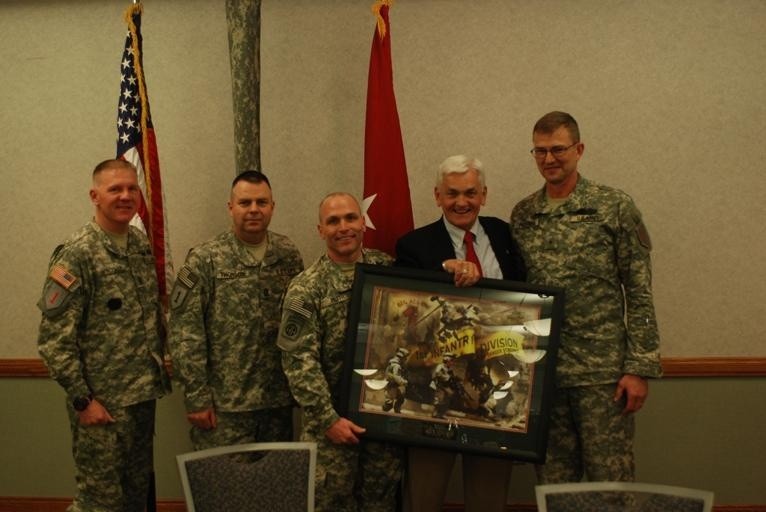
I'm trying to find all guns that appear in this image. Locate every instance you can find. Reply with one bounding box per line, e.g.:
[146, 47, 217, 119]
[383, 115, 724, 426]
[447, 369, 474, 406]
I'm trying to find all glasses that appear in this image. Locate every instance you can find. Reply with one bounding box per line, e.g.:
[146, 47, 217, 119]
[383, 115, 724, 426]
[531, 141, 576, 159]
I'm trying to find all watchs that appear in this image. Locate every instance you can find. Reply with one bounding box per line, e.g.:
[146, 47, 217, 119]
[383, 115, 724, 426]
[73, 393, 93, 411]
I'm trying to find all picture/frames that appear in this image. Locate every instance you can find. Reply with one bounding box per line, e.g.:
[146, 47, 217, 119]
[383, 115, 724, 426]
[329, 262, 567, 468]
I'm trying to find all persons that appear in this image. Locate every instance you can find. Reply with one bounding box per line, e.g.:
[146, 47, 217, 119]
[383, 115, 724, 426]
[274, 190, 481, 510]
[382, 343, 409, 414]
[36, 157, 166, 512]
[478, 363, 500, 417]
[168, 170, 304, 453]
[430, 351, 454, 418]
[395, 154, 544, 512]
[507, 109, 668, 512]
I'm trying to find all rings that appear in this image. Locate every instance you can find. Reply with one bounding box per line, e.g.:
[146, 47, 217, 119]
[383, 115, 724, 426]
[463, 269, 468, 274]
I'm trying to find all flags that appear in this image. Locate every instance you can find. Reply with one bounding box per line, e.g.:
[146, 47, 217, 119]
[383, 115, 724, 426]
[355, 0, 415, 259]
[113, 4, 164, 302]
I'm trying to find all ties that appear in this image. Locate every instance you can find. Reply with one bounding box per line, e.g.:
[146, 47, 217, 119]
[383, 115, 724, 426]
[463, 230, 484, 278]
